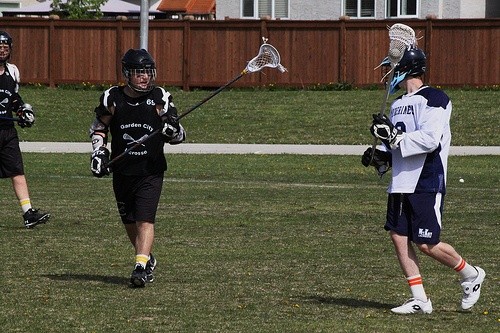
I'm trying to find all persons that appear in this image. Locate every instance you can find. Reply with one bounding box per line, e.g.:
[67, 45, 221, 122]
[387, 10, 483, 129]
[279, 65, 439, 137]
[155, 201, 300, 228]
[0, 31, 50, 229]
[362, 46, 486, 314]
[89, 48, 186, 288]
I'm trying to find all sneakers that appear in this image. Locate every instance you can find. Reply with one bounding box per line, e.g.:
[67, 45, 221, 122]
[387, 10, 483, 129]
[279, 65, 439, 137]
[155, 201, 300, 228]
[146, 252, 157, 283]
[460, 266, 486, 309]
[22, 207, 51, 229]
[131, 263, 147, 289]
[390, 298, 433, 315]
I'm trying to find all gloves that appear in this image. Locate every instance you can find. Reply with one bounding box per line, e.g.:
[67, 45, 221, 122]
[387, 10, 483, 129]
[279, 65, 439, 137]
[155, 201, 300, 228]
[16, 103, 35, 128]
[90, 146, 110, 178]
[159, 115, 183, 145]
[361, 147, 391, 179]
[370, 112, 403, 151]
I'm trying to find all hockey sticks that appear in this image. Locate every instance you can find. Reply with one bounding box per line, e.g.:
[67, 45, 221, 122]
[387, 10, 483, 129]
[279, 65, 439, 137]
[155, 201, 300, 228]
[371, 22, 424, 163]
[0, 116, 20, 122]
[106, 37, 289, 169]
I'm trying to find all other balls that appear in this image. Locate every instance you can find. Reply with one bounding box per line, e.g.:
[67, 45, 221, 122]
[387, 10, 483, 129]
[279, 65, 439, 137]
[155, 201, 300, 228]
[390, 48, 401, 59]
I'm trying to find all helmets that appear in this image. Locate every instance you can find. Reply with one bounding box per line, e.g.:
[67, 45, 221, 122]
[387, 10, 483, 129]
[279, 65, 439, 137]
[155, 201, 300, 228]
[122, 48, 157, 93]
[0, 31, 13, 65]
[381, 47, 427, 93]
